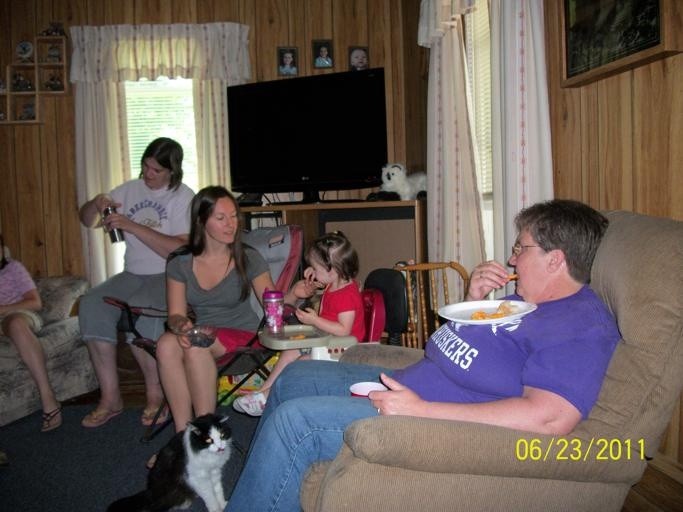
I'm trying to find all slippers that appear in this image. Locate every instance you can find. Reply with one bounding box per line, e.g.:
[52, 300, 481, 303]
[42, 408, 61, 432]
[142, 405, 169, 425]
[82, 410, 122, 428]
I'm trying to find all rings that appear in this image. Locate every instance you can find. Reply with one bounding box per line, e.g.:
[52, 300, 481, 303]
[378, 408, 381, 413]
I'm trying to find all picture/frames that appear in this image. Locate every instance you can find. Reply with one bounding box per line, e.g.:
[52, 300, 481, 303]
[557, 0, 683, 88]
[276, 46, 299, 77]
[310, 40, 334, 69]
[346, 45, 369, 71]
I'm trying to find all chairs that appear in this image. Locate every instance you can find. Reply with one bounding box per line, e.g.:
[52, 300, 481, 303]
[258, 290, 385, 363]
[361, 268, 410, 346]
[101, 225, 305, 453]
[395, 262, 471, 349]
[299, 207, 683, 512]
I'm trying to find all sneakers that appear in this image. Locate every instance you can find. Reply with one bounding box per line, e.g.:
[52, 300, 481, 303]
[233, 392, 266, 416]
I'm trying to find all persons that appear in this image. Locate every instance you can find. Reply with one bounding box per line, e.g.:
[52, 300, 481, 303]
[232, 229, 366, 417]
[76, 137, 196, 428]
[146, 185, 325, 471]
[350, 49, 368, 71]
[222, 199, 621, 511]
[279, 52, 297, 75]
[314, 46, 333, 68]
[0, 234, 62, 432]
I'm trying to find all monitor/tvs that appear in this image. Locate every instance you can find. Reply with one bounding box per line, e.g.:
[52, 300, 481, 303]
[227, 67, 388, 206]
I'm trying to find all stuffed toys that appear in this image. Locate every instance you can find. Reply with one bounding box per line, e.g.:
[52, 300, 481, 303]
[382, 165, 426, 202]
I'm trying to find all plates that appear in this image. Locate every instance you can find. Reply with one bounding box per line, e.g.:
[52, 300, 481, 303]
[437, 299, 538, 325]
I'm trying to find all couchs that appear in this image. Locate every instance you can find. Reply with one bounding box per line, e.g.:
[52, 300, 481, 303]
[0, 276, 101, 427]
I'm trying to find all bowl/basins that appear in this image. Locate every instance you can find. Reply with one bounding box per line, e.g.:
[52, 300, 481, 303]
[184, 324, 217, 348]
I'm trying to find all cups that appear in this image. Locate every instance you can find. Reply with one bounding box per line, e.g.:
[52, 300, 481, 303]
[262, 290, 283, 335]
[347, 381, 389, 399]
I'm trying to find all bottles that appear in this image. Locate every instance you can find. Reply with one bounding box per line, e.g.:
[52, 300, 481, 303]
[102, 206, 125, 244]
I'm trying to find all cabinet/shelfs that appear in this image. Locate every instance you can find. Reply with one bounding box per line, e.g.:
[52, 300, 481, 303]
[237, 200, 427, 347]
[0, 36, 70, 124]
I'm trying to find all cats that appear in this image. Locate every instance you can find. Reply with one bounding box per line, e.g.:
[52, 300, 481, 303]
[107, 413, 236, 512]
